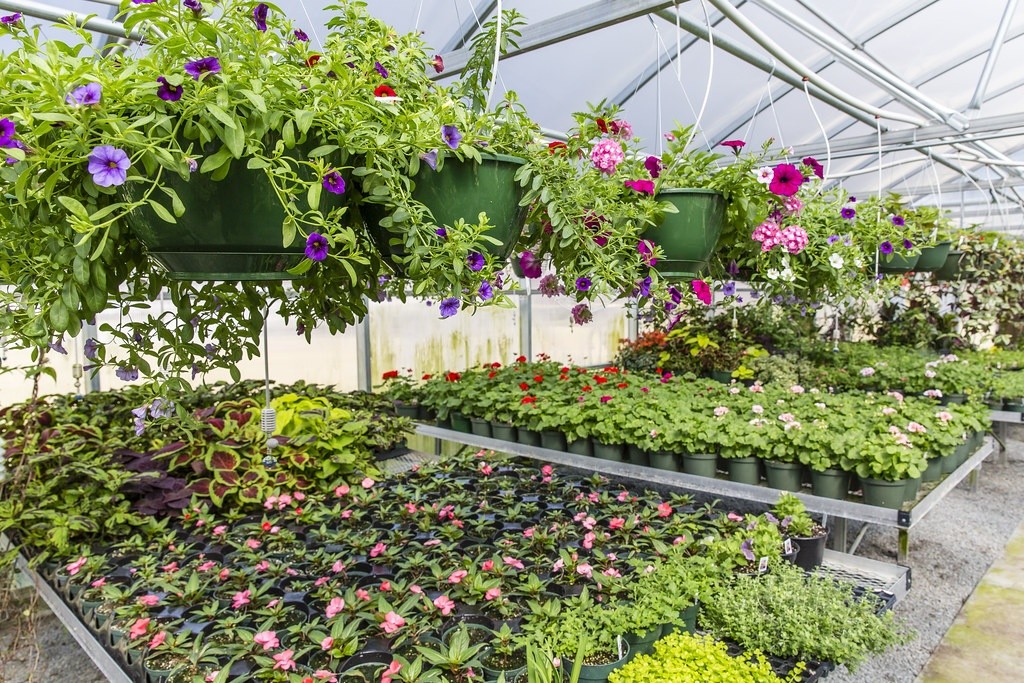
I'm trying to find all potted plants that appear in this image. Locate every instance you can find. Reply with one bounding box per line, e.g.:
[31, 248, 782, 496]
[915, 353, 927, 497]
[485, 382, 517, 443]
[719, 422, 763, 485]
[1001, 388, 1024, 420]
[984, 383, 1005, 410]
[559, 402, 592, 456]
[808, 429, 851, 498]
[535, 395, 561, 448]
[960, 406, 978, 452]
[468, 391, 492, 438]
[93, 580, 145, 628]
[478, 622, 536, 682]
[305, 548, 349, 580]
[538, 507, 573, 530]
[706, 343, 743, 383]
[972, 398, 991, 445]
[686, 417, 719, 480]
[554, 589, 630, 683]
[465, 514, 504, 542]
[620, 403, 650, 467]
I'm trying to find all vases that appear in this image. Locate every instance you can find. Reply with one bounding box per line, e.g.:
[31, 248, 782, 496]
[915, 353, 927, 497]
[918, 451, 942, 482]
[940, 440, 972, 472]
[517, 425, 537, 447]
[878, 241, 920, 275]
[940, 250, 973, 282]
[649, 450, 683, 472]
[662, 599, 703, 644]
[622, 619, 665, 663]
[901, 474, 921, 502]
[393, 400, 418, 419]
[449, 412, 472, 434]
[790, 520, 832, 570]
[763, 458, 805, 490]
[114, 128, 349, 283]
[918, 238, 956, 271]
[638, 190, 726, 272]
[940, 391, 966, 406]
[360, 154, 535, 281]
[860, 474, 907, 510]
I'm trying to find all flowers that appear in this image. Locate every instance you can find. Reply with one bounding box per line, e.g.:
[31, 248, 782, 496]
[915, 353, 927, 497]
[0, 0, 1024, 683]
